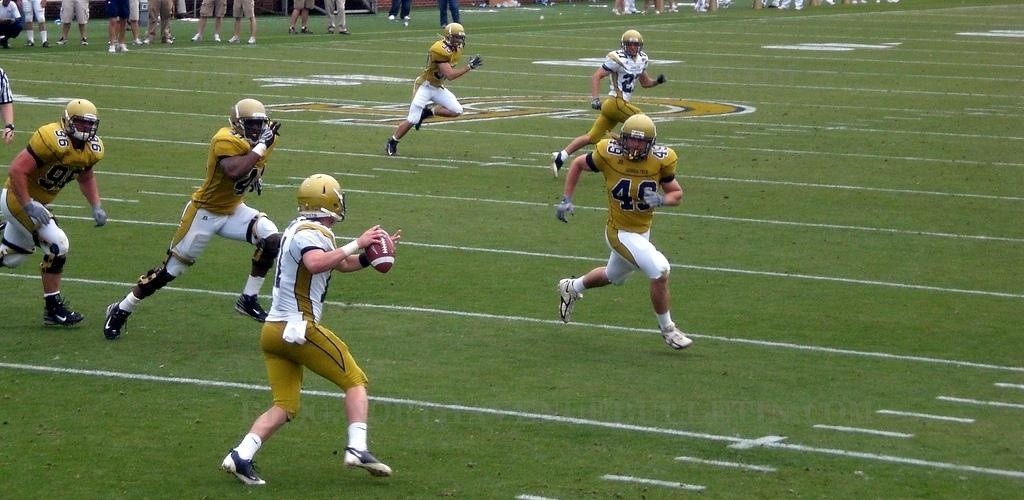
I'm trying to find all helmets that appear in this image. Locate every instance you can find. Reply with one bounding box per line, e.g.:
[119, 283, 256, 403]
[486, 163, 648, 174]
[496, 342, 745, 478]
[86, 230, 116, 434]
[231, 99, 267, 140]
[297, 174, 345, 222]
[444, 23, 465, 48]
[620, 30, 643, 55]
[64, 99, 99, 141]
[619, 114, 657, 160]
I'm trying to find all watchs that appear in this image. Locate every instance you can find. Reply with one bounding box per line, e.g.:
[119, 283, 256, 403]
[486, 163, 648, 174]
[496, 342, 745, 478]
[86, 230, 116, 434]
[5, 123, 15, 131]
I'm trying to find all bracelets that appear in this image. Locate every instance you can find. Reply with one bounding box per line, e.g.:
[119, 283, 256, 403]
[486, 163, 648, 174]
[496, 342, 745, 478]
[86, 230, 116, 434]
[341, 242, 358, 254]
[251, 143, 267, 156]
[357, 252, 369, 269]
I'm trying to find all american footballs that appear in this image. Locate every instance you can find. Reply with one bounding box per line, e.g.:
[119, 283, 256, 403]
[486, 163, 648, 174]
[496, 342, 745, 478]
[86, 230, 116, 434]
[363, 230, 395, 274]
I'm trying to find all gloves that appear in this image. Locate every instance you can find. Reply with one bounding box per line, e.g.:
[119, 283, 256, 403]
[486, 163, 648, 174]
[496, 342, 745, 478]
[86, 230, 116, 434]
[657, 74, 666, 84]
[644, 190, 663, 207]
[557, 197, 573, 223]
[591, 98, 601, 110]
[93, 205, 106, 227]
[24, 202, 50, 226]
[259, 121, 280, 148]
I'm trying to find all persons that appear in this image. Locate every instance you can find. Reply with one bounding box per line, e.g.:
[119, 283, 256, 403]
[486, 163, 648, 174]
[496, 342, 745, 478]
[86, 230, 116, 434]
[694, 0, 735, 13]
[191, 0, 227, 42]
[219, 174, 402, 487]
[551, 29, 669, 177]
[384, 22, 482, 157]
[0, 69, 16, 145]
[104, 0, 175, 53]
[438, 1, 464, 29]
[388, 1, 412, 21]
[554, 112, 696, 351]
[612, 0, 678, 15]
[1, 0, 52, 48]
[751, 0, 901, 10]
[228, 2, 257, 44]
[289, 1, 314, 34]
[56, 1, 90, 45]
[101, 98, 282, 342]
[478, 2, 521, 8]
[0, 96, 106, 330]
[324, 1, 350, 35]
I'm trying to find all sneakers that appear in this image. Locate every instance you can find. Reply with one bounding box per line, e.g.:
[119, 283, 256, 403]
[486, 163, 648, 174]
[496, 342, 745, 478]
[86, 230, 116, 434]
[551, 152, 563, 177]
[44, 302, 132, 340]
[344, 446, 391, 477]
[222, 448, 266, 485]
[107, 38, 174, 52]
[192, 34, 256, 44]
[27, 37, 88, 47]
[289, 26, 350, 34]
[663, 324, 692, 349]
[235, 294, 268, 323]
[558, 278, 583, 323]
[386, 108, 433, 156]
